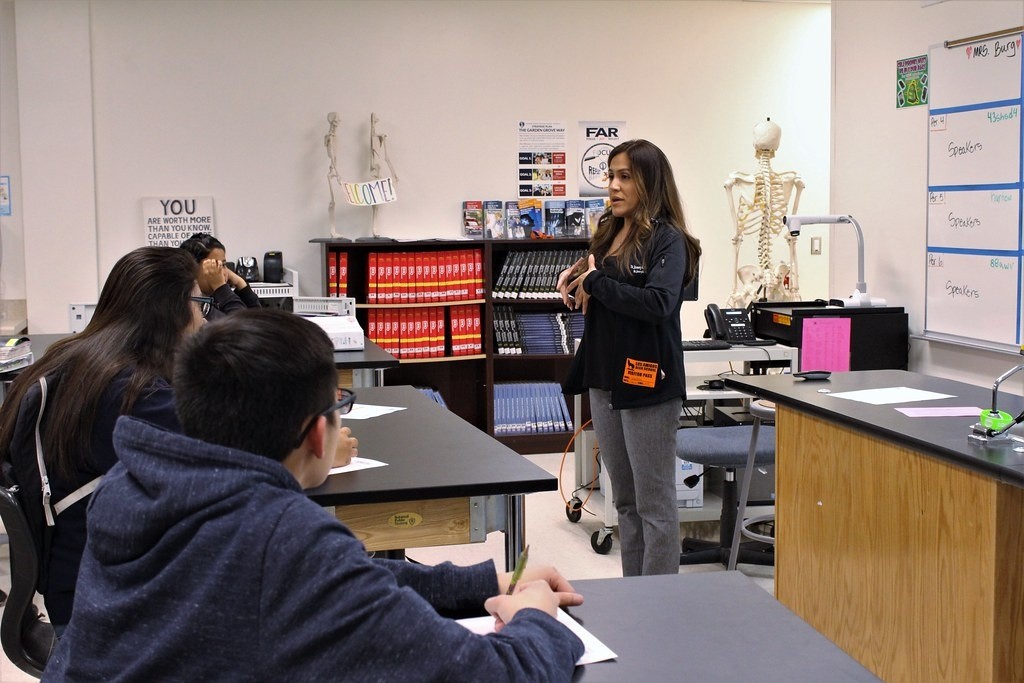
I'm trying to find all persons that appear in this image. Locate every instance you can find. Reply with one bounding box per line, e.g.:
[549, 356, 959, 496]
[0, 247, 358, 641]
[555, 140, 702, 577]
[534, 155, 551, 196]
[179, 232, 261, 321]
[40, 310, 585, 683]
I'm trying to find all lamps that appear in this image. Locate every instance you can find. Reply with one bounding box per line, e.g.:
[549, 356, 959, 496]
[783, 214, 888, 309]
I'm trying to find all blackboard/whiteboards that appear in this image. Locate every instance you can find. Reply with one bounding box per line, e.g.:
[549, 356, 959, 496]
[910, 24, 1024, 357]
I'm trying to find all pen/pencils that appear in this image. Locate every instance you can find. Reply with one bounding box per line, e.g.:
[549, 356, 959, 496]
[506, 544, 529, 596]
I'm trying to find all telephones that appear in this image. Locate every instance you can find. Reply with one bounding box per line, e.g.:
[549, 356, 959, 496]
[704, 304, 756, 342]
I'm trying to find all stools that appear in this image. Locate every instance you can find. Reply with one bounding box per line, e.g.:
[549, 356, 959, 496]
[726, 398, 777, 574]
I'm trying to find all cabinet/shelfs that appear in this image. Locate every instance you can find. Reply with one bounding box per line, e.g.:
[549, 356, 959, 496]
[321, 236, 593, 456]
[567, 301, 911, 555]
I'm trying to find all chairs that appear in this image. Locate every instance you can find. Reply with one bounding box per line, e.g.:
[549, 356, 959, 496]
[673, 425, 778, 566]
[0, 478, 56, 680]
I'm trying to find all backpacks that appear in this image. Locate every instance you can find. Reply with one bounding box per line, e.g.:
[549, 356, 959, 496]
[10, 372, 106, 595]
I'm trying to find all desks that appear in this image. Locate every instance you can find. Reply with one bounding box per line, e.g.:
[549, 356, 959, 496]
[0, 299, 77, 387]
[452, 567, 884, 683]
[332, 337, 398, 386]
[723, 368, 1023, 682]
[307, 392, 558, 573]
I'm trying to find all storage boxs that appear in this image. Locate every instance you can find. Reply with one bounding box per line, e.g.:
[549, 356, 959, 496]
[599, 421, 703, 507]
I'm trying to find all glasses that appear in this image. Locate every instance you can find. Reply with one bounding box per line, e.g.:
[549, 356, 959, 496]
[191, 296, 214, 317]
[295, 388, 356, 451]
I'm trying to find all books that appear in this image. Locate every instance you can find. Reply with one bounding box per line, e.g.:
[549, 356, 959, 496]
[493, 382, 574, 435]
[492, 305, 585, 355]
[328, 252, 348, 298]
[492, 250, 588, 300]
[368, 249, 484, 359]
[417, 389, 447, 409]
[462, 199, 606, 239]
